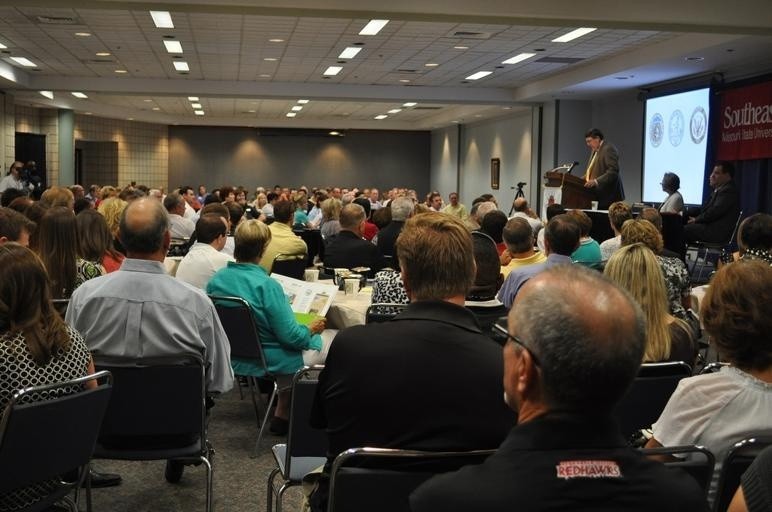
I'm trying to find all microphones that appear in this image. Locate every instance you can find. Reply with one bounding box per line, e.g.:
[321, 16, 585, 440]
[566, 160, 579, 173]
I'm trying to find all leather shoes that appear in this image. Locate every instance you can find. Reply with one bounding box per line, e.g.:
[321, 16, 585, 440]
[65, 466, 122, 488]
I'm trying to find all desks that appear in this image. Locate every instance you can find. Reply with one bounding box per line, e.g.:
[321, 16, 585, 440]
[564, 206, 641, 245]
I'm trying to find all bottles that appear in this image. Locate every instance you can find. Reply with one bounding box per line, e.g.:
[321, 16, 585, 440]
[246, 209, 253, 219]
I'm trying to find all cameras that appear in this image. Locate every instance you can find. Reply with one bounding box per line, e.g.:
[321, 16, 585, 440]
[516, 182, 526, 188]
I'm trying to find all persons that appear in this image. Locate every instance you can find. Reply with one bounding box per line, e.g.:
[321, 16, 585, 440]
[1, 157, 772, 512]
[580, 127, 623, 209]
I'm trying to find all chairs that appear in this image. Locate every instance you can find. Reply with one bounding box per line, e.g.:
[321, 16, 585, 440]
[683, 209, 743, 285]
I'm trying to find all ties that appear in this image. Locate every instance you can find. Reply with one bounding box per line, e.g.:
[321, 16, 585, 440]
[585, 152, 598, 181]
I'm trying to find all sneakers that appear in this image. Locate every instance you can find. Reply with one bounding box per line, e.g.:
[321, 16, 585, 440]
[268, 416, 289, 434]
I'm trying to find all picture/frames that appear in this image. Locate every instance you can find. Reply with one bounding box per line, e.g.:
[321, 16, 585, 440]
[490, 158, 500, 189]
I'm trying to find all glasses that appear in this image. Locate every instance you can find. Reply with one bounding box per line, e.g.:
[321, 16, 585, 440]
[490, 317, 544, 367]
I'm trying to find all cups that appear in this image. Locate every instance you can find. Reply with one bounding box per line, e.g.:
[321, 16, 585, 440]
[592, 201, 599, 211]
[333, 266, 371, 296]
[305, 270, 320, 281]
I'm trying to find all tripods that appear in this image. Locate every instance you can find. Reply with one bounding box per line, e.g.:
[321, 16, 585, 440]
[509, 188, 525, 217]
